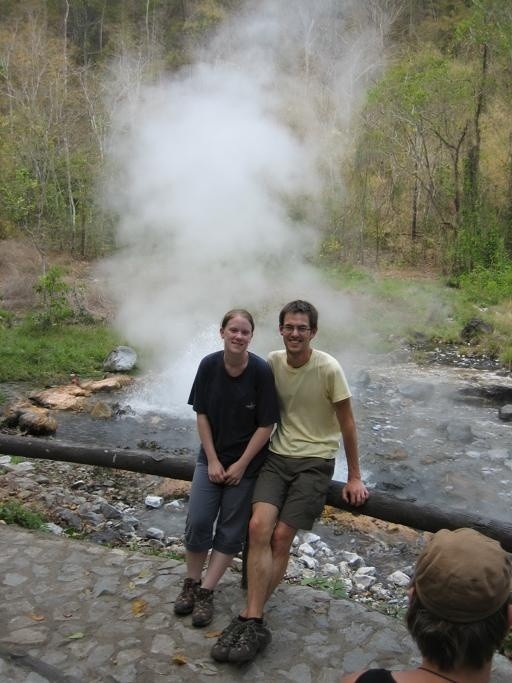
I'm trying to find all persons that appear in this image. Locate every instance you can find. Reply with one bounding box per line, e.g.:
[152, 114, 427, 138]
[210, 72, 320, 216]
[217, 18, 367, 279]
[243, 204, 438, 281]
[211, 300, 370, 667]
[174, 308, 279, 629]
[337, 528, 511, 682]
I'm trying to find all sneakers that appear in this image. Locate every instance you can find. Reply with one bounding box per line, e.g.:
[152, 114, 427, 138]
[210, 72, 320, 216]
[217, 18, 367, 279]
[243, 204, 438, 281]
[175, 577, 202, 615]
[228, 618, 271, 665]
[192, 585, 214, 627]
[211, 617, 247, 663]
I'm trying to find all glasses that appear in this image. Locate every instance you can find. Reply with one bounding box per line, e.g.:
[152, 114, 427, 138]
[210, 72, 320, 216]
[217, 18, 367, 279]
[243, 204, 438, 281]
[282, 325, 312, 332]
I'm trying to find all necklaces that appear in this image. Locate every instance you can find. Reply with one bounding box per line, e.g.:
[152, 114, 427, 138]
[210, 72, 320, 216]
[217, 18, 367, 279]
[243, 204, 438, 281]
[419, 667, 455, 682]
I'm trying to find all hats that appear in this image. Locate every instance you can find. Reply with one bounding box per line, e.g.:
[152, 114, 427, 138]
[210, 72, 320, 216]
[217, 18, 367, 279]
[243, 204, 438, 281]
[413, 528, 512, 622]
